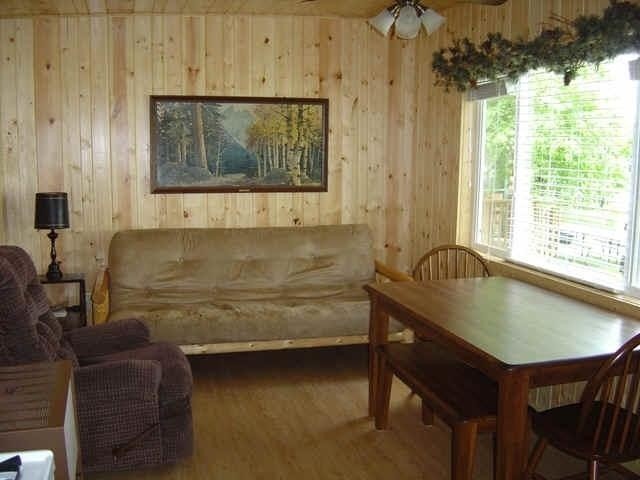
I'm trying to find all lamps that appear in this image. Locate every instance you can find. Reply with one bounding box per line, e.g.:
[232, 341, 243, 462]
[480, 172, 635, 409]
[32, 192, 69, 274]
[366, 1, 448, 40]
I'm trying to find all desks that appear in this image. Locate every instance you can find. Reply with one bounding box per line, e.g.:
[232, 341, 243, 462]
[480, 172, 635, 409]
[36, 274, 87, 327]
[361, 273, 640, 479]
[0, 450, 55, 480]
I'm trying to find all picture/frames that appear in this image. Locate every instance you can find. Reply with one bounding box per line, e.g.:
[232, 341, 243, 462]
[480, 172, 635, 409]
[150, 91, 330, 194]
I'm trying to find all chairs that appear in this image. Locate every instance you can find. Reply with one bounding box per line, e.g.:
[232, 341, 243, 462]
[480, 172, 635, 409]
[411, 245, 495, 426]
[0, 244, 195, 474]
[525, 335, 640, 479]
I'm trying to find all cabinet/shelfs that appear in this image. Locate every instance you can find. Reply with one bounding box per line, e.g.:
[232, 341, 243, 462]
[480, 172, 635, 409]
[0, 363, 86, 480]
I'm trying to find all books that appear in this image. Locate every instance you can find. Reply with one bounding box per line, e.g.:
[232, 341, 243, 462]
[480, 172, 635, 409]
[52, 309, 68, 318]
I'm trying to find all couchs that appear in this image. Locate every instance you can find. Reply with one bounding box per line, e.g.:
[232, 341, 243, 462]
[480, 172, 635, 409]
[93, 224, 413, 357]
